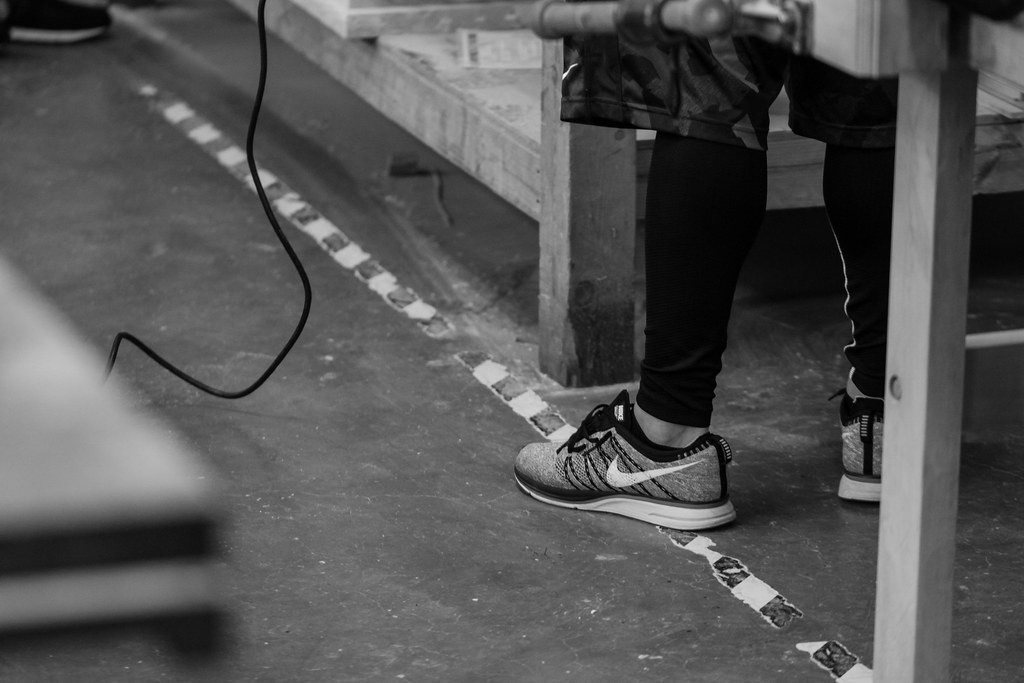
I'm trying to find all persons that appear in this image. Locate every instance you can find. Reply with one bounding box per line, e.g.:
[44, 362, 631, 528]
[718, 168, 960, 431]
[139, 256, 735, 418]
[513, 1, 917, 531]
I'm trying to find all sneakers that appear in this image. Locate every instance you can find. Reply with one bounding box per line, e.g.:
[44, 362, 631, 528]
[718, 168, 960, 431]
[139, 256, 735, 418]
[515, 389, 737, 530]
[827, 387, 884, 501]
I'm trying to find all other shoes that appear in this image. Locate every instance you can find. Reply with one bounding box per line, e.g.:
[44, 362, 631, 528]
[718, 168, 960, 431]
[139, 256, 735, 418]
[9, 0, 111, 43]
